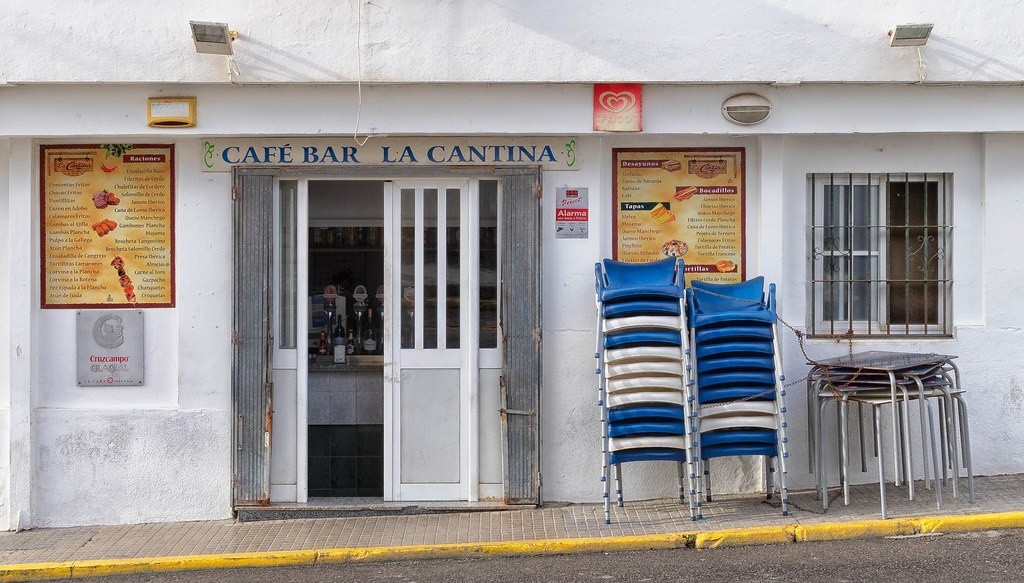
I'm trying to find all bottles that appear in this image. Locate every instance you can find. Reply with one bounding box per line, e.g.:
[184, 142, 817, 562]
[346, 330, 355, 355]
[309, 227, 383, 247]
[319, 333, 327, 355]
[363, 309, 377, 354]
[335, 315, 345, 345]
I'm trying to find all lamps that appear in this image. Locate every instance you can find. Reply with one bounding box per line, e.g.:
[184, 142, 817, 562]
[890, 22, 936, 47]
[721, 92, 772, 127]
[191, 20, 238, 57]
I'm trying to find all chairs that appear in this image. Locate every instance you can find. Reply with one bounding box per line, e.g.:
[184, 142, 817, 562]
[595, 256, 792, 523]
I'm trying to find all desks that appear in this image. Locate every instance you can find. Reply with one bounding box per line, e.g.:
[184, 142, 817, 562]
[807, 347, 975, 520]
